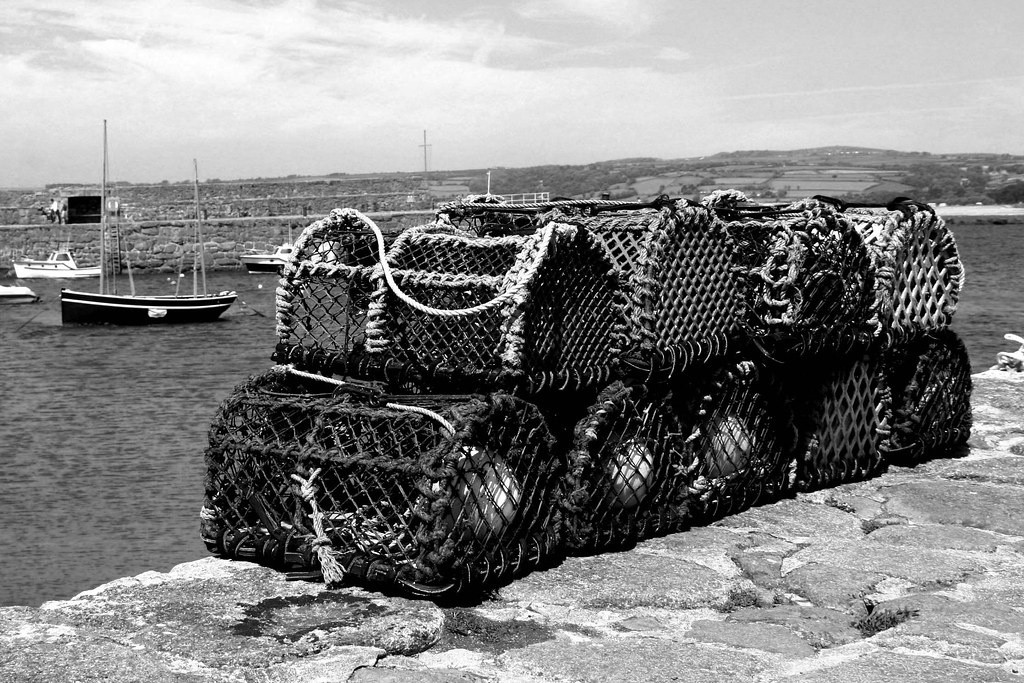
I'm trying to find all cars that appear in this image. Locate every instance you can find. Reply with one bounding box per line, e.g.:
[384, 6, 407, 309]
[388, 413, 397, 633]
[0, 284, 38, 306]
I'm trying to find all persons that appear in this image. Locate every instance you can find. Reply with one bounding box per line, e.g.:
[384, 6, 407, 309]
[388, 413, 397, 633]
[59, 200, 68, 225]
[50, 198, 61, 224]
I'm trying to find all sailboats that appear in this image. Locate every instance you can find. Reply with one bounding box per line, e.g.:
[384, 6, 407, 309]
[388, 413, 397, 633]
[61, 119, 238, 325]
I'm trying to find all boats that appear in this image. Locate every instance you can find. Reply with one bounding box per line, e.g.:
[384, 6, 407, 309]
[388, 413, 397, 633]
[240, 246, 295, 276]
[26, 267, 101, 280]
[12, 249, 102, 278]
